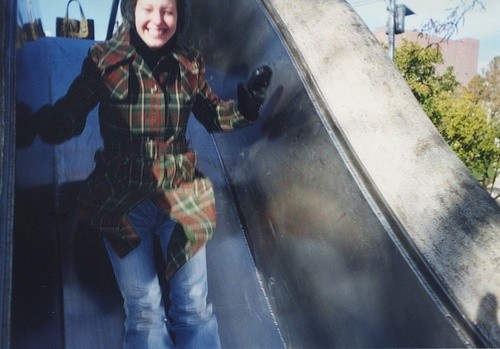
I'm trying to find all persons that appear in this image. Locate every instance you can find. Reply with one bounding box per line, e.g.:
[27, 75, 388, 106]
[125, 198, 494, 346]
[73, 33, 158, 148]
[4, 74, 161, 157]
[20, 0, 278, 349]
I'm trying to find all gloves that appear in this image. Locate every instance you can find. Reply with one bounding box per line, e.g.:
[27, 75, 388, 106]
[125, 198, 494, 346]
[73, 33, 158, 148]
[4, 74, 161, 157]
[237, 64, 272, 119]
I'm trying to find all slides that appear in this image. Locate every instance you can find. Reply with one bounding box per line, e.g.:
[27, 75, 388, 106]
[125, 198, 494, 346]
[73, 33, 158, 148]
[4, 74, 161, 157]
[0, 0, 500, 349]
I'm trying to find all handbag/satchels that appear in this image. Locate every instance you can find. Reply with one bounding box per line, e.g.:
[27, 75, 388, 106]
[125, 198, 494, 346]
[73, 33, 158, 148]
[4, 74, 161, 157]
[55, 0, 95, 41]
